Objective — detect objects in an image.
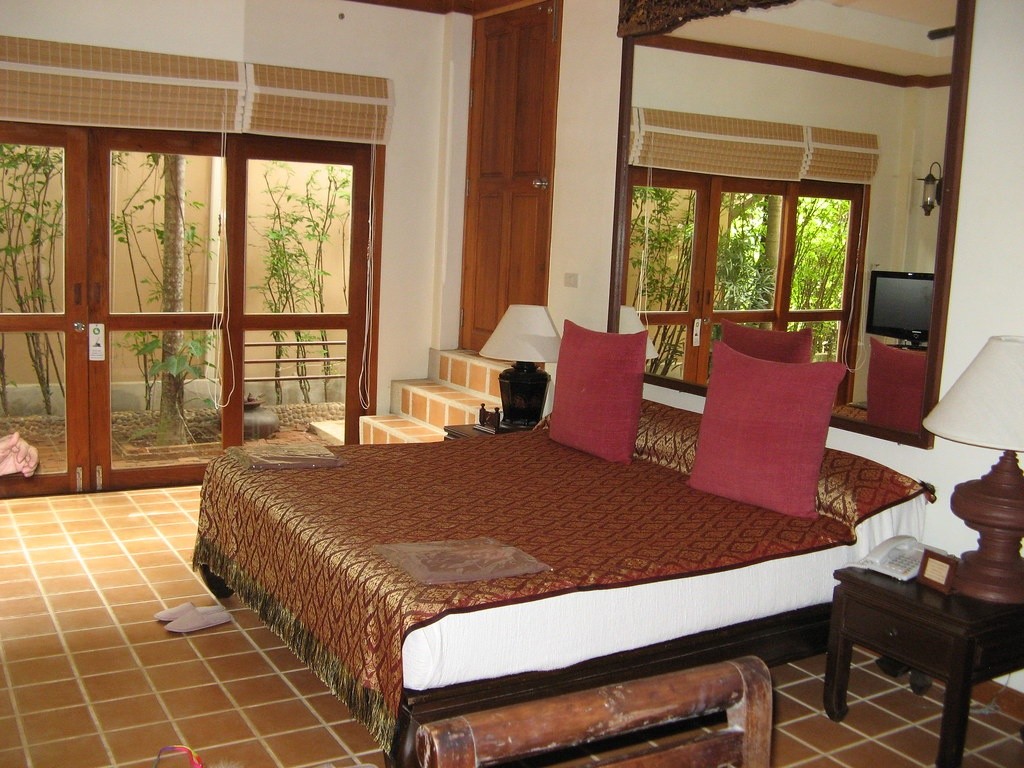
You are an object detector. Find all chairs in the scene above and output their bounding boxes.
[414,655,775,768]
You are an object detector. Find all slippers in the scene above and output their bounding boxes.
[163,608,234,633]
[154,601,226,621]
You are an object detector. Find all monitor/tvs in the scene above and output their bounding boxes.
[865,269,935,351]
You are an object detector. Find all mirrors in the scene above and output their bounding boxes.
[604,0,975,451]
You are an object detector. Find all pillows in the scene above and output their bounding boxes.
[718,316,814,363]
[867,334,926,434]
[548,318,648,469]
[685,339,847,521]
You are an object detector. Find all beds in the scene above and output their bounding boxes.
[194,397,938,768]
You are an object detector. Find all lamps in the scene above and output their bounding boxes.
[923,333,1024,604]
[478,303,561,429]
[916,160,942,218]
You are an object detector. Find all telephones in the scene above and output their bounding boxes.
[862,535,948,582]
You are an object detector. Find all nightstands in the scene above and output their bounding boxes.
[443,424,509,440]
[822,563,1023,768]
